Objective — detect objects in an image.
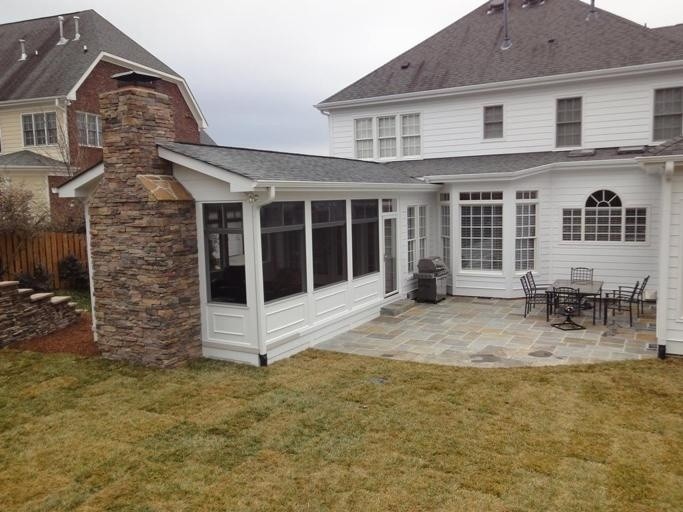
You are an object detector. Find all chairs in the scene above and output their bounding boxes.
[519,267,649,331]
